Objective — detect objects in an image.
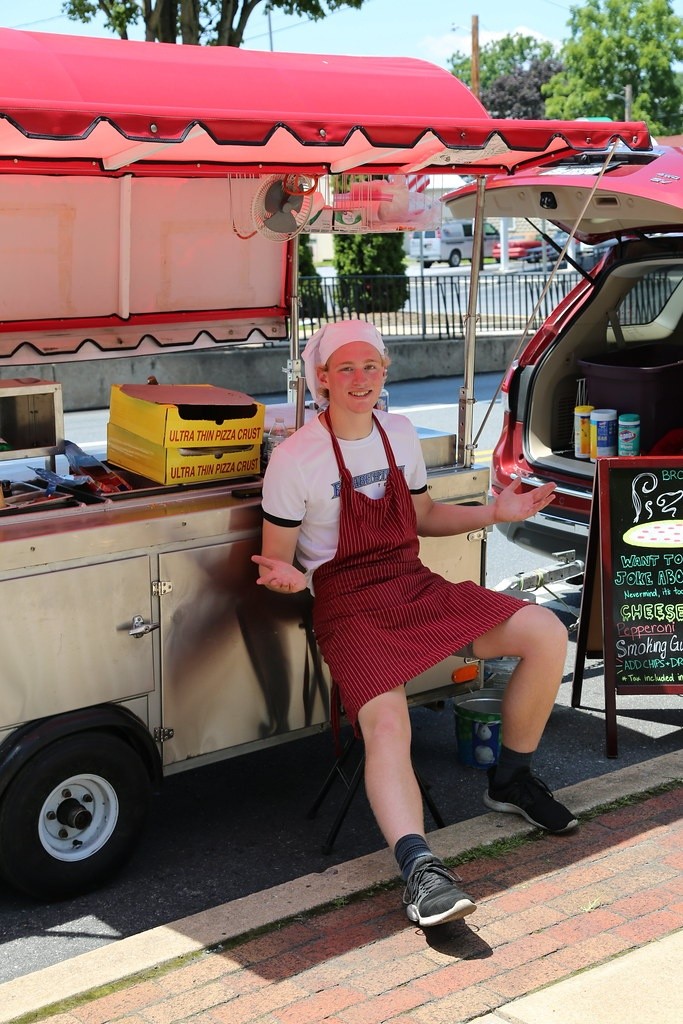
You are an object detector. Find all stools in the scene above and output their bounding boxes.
[308,672,447,855]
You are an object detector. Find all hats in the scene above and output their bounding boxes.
[301,319,385,411]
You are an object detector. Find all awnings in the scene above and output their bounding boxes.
[0,97,652,447]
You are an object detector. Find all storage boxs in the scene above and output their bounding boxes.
[107,421,261,486]
[111,382,265,448]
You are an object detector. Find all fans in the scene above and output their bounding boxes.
[250,174,313,243]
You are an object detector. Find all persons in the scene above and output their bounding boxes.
[251,318,579,927]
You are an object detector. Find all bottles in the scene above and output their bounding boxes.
[265,417,289,469]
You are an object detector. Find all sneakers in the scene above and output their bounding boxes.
[401,855,476,926]
[482,766,579,833]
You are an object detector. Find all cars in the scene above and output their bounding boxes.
[437,133,682,561]
[543,227,582,259]
[578,233,648,256]
[493,232,553,263]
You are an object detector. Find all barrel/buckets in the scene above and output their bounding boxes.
[452,688,508,769]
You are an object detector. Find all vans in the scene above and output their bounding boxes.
[409,219,500,269]
